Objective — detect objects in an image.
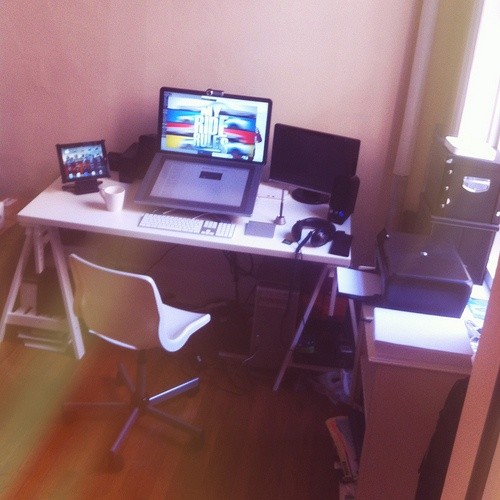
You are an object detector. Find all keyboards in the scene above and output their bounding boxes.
[137,210,237,239]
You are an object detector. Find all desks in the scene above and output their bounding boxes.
[0,166,361,392]
[323,269,493,500]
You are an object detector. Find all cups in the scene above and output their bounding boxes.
[99,186,125,211]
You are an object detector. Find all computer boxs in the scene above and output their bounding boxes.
[247,258,314,374]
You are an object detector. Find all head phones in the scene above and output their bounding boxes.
[291,217,337,248]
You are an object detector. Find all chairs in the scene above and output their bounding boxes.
[55,253,211,468]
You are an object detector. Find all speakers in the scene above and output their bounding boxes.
[327,175,361,225]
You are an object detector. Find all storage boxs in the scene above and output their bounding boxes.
[417,194,500,284]
[425,150,500,224]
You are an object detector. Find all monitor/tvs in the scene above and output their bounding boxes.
[56,139,112,196]
[267,123,361,204]
[156,87,275,171]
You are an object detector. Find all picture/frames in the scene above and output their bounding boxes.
[57,139,109,180]
